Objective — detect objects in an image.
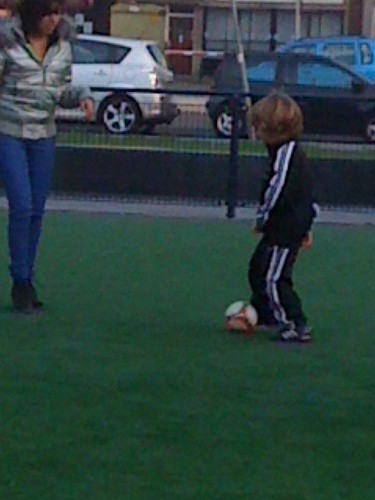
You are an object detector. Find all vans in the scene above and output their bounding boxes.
[273,37,375,83]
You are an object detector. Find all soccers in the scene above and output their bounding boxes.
[221,300,257,332]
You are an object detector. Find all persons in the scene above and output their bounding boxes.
[0,0,96,315]
[249,90,321,340]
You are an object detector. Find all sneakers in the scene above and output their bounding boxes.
[270,329,311,343]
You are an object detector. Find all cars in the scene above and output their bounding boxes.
[205,50,374,144]
[54,34,180,133]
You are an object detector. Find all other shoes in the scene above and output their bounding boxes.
[11,284,42,315]
